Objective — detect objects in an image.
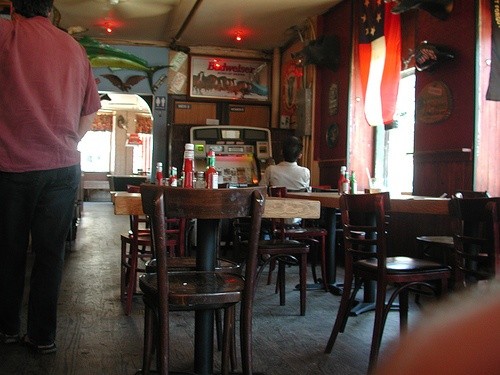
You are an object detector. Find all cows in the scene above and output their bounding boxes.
[286,16,342,71]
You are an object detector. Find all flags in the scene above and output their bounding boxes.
[358,0,401,126]
[486,0,500,101]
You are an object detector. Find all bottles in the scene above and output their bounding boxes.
[195,151,218,189]
[338,166,357,195]
[182,144,194,188]
[156,162,177,187]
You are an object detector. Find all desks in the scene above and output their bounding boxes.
[288,192,381,294]
[115,192,320,375]
[348,194,452,316]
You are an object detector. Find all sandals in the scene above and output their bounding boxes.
[22,334,57,354]
[0,334,19,346]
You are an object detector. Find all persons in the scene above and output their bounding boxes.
[259,136,310,228]
[0,0,102,354]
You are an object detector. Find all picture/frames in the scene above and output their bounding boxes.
[278,32,307,130]
[188,53,272,105]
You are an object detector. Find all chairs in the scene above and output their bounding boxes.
[121,166,500,375]
